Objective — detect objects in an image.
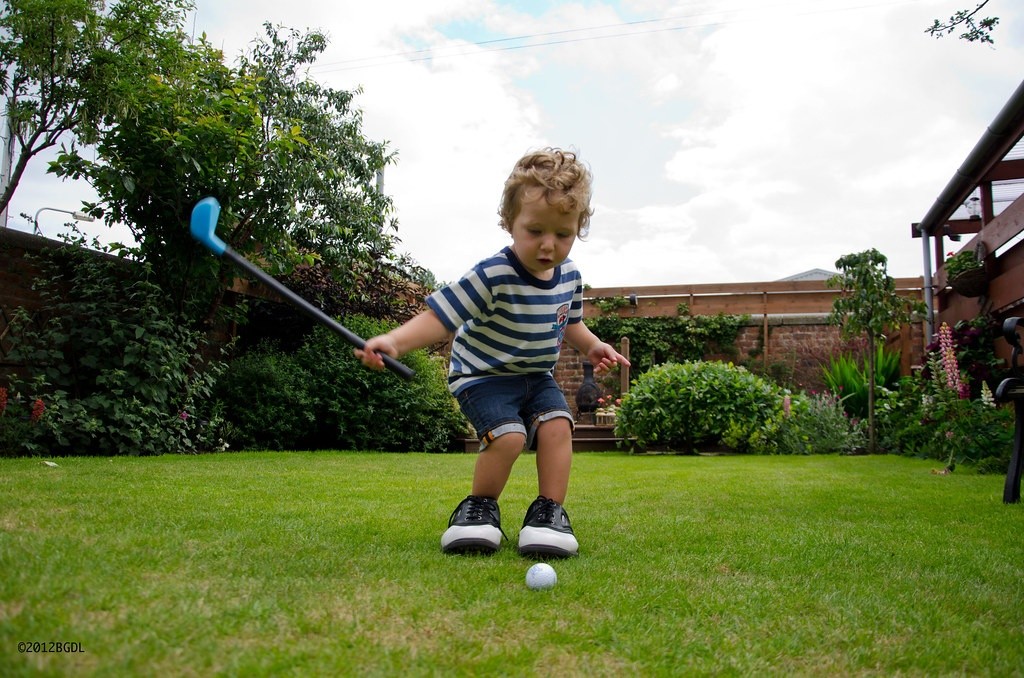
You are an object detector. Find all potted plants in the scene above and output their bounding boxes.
[947,250,988,297]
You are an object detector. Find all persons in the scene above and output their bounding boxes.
[353,147,631,559]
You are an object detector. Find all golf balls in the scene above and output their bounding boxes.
[523,562,557,593]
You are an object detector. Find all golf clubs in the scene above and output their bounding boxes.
[188,197,417,385]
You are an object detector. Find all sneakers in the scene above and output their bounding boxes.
[518,498,578,557]
[440,497,502,552]
